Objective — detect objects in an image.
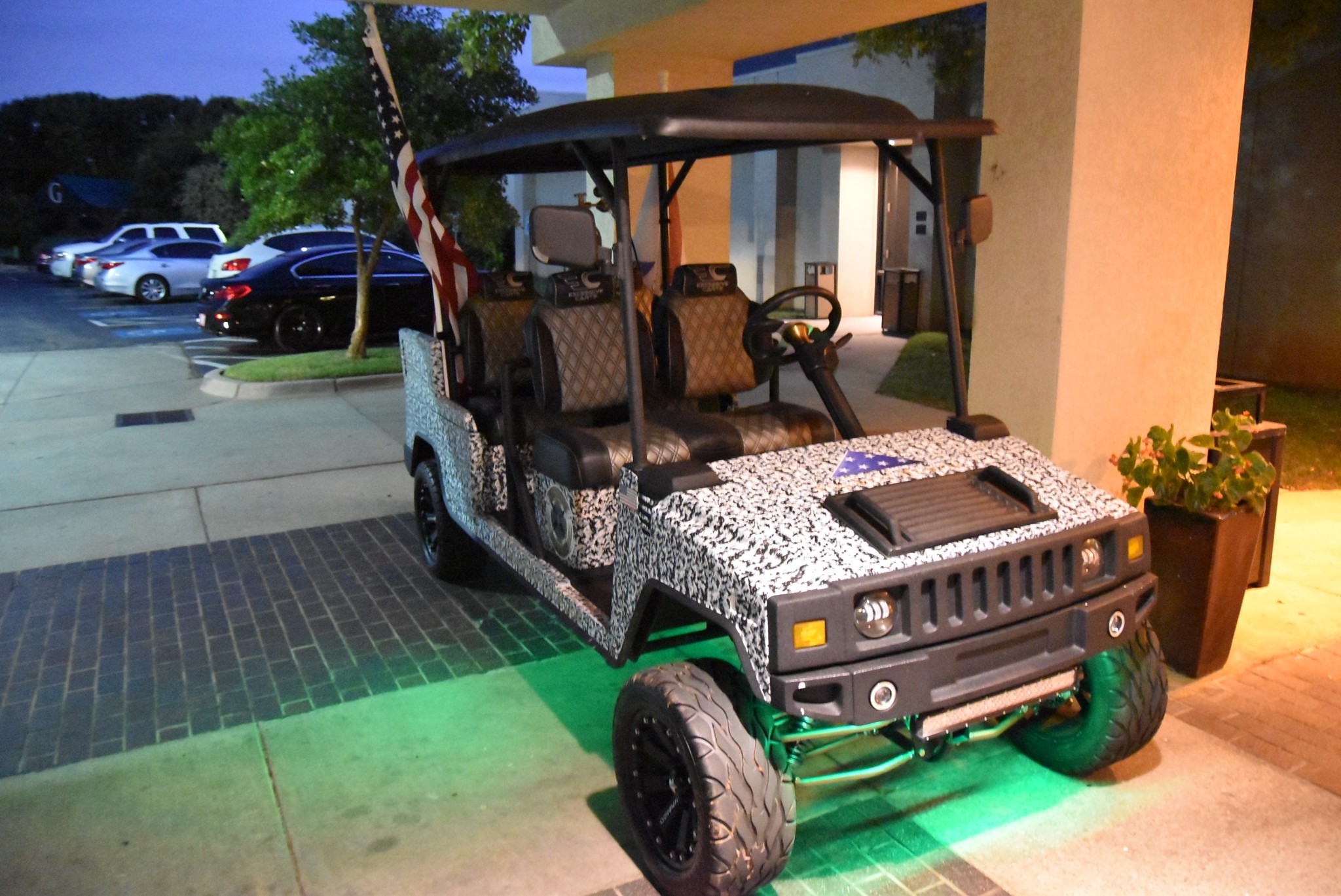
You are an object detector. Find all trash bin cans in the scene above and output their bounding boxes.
[879,268,920,333]
[804,261,835,318]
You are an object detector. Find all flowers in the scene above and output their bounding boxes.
[1109,407,1277,518]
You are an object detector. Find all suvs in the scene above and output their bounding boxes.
[51,223,228,286]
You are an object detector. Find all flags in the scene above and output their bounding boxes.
[361,4,485,323]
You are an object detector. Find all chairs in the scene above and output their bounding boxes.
[524,269,714,490]
[651,264,835,461]
[612,271,657,377]
[458,272,537,449]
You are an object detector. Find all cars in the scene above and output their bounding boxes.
[37,249,51,273]
[75,240,148,287]
[195,243,436,354]
[98,239,225,303]
[206,224,404,279]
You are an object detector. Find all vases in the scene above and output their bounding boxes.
[1144,494,1264,678]
